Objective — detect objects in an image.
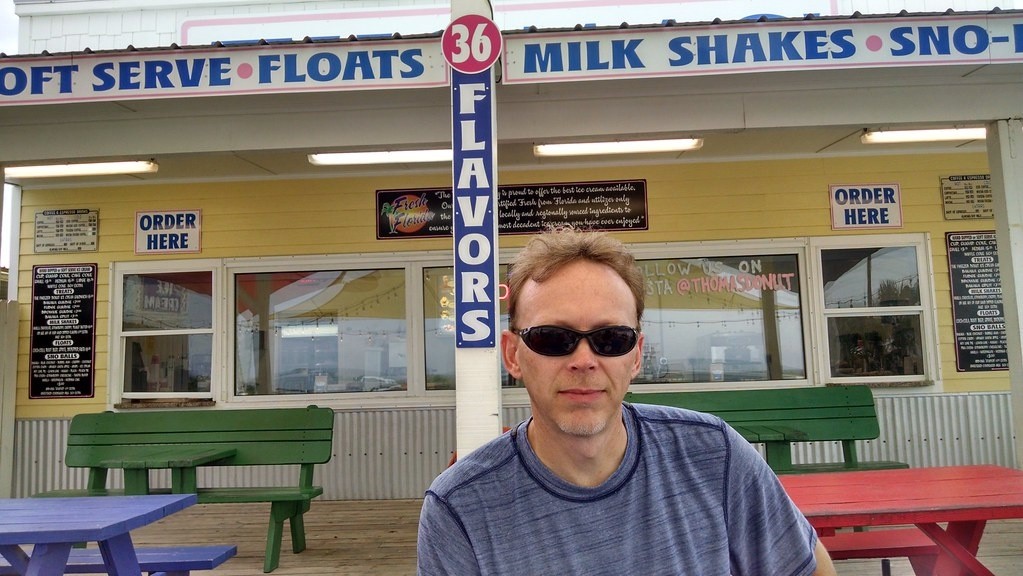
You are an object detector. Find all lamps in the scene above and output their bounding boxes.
[534,139,704,157]
[860,127,987,144]
[307,148,453,167]
[5,160,159,178]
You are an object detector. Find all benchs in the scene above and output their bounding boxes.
[623,383,909,534]
[30,405,336,573]
[820,528,946,576]
[0,545,237,576]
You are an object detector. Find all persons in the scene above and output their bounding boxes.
[416,222,836,576]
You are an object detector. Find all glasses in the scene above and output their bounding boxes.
[512,325,640,357]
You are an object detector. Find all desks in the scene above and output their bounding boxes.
[99,447,236,496]
[0,494,198,576]
[777,465,1023,576]
[730,423,808,473]
